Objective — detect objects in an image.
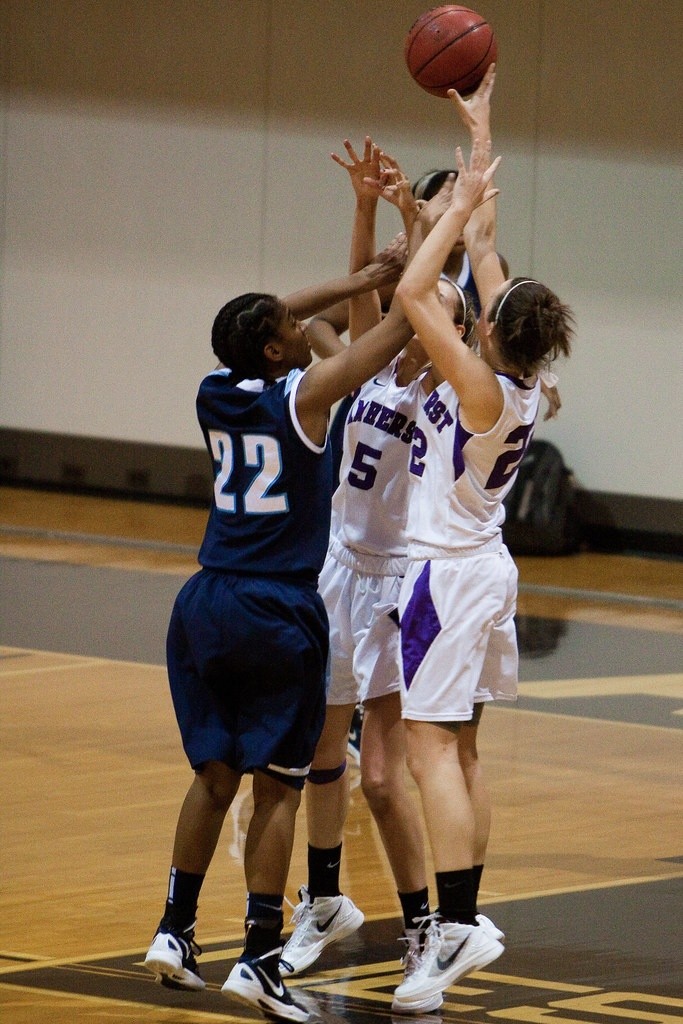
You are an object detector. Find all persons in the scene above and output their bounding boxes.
[309,169,562,767]
[395,63,571,1003]
[278,134,474,976]
[144,173,462,1022]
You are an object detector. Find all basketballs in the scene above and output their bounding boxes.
[405,4,499,98]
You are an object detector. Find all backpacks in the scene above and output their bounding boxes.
[501,440,569,550]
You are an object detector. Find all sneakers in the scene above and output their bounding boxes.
[346,703,364,763]
[278,885,364,976]
[474,914,504,940]
[221,959,324,1024]
[390,929,443,1013]
[144,925,206,991]
[394,912,505,1002]
